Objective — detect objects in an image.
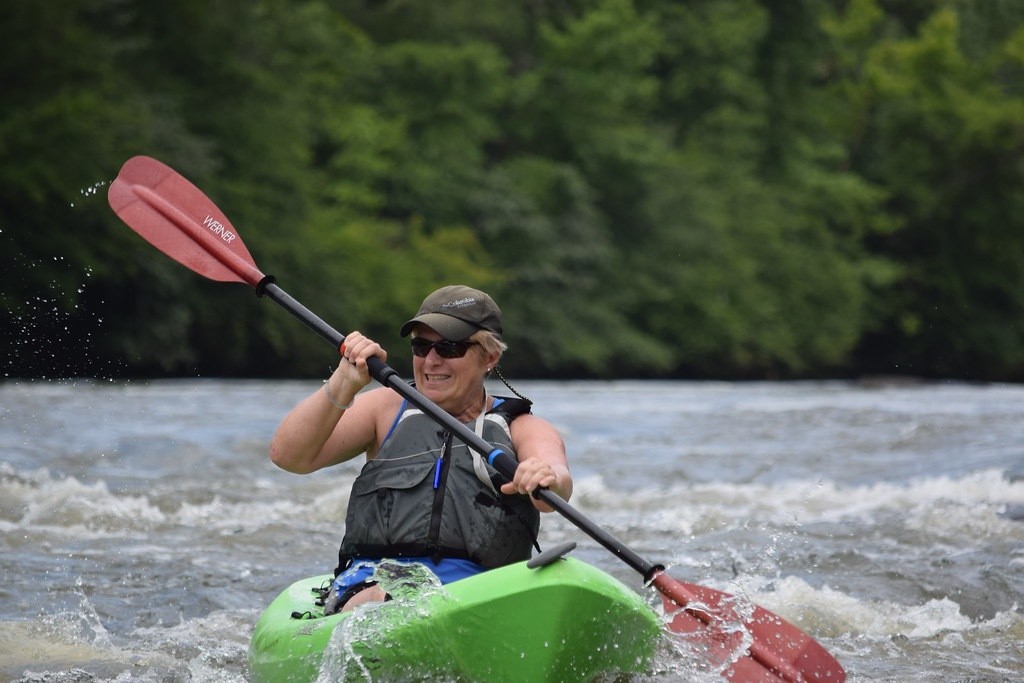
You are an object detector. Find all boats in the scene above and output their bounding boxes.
[247,541,666,683]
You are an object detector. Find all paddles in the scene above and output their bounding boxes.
[107,155,847,683]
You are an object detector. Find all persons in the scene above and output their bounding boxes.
[270,286,572,616]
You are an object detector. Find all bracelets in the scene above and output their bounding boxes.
[323,380,354,410]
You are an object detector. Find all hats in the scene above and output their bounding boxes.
[401,285,503,343]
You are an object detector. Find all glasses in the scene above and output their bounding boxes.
[409,338,480,359]
